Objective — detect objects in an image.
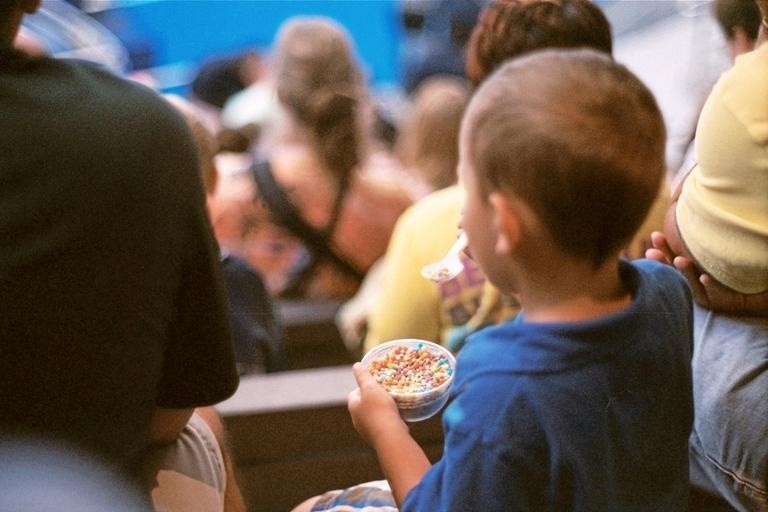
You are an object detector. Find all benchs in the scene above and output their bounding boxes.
[220,363,442,512]
[266,294,365,372]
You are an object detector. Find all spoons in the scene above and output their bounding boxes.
[421,230,469,283]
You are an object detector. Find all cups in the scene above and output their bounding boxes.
[360,338,456,423]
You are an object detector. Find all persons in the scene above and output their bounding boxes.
[1,1,768,512]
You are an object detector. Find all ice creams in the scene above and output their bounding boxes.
[369,341,453,422]
